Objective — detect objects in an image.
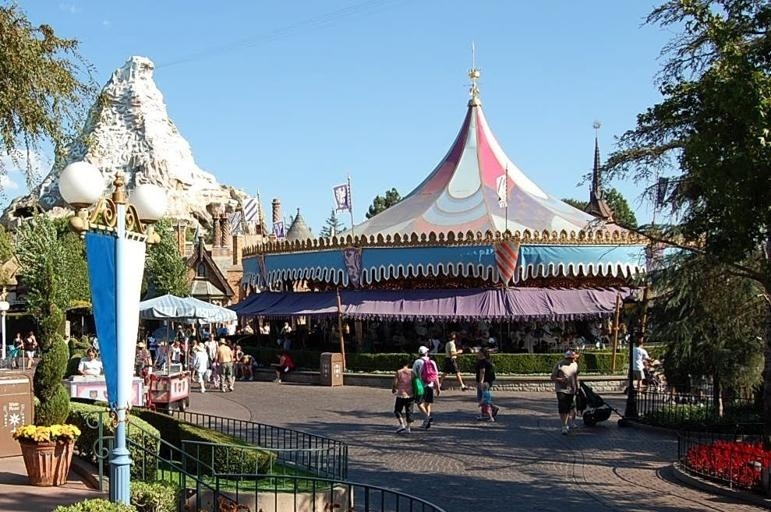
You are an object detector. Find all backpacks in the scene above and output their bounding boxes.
[420,358,437,382]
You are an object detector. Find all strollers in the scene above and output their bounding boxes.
[0,349,20,369]
[579,381,625,426]
[642,362,659,392]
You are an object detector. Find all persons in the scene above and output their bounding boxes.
[440,331,471,391]
[316,316,627,353]
[568,396,578,428]
[392,358,415,434]
[475,348,499,417]
[551,349,579,435]
[78,348,103,376]
[135,322,295,394]
[411,346,441,428]
[477,382,495,422]
[628,336,653,390]
[7,330,38,369]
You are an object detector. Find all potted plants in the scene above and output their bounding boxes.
[10,198,83,488]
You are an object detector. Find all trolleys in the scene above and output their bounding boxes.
[60,363,188,415]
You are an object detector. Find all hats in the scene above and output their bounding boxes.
[197,343,206,351]
[136,342,146,347]
[565,351,579,358]
[218,338,227,344]
[418,346,429,354]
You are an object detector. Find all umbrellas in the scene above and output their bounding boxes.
[139,291,220,376]
[182,295,238,325]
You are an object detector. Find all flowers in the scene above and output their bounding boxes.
[11,424,83,444]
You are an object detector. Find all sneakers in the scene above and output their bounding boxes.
[396,425,410,434]
[562,424,570,434]
[423,416,433,427]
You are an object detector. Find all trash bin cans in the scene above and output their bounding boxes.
[1,369,35,456]
[321,352,343,386]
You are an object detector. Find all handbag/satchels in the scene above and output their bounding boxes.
[414,376,424,402]
